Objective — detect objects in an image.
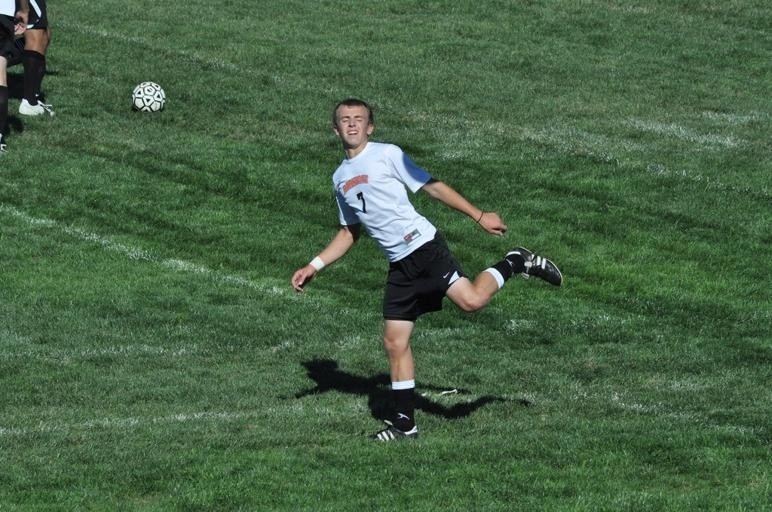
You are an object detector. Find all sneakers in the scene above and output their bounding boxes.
[505,246,563,287]
[18,98,56,117]
[368,424,418,445]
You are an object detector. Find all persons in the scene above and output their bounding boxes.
[289,98,564,445]
[1,0,30,152]
[7,0,56,118]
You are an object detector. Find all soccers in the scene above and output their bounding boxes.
[132,81,166,114]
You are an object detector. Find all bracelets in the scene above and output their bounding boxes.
[309,256,326,272]
[475,211,484,224]
